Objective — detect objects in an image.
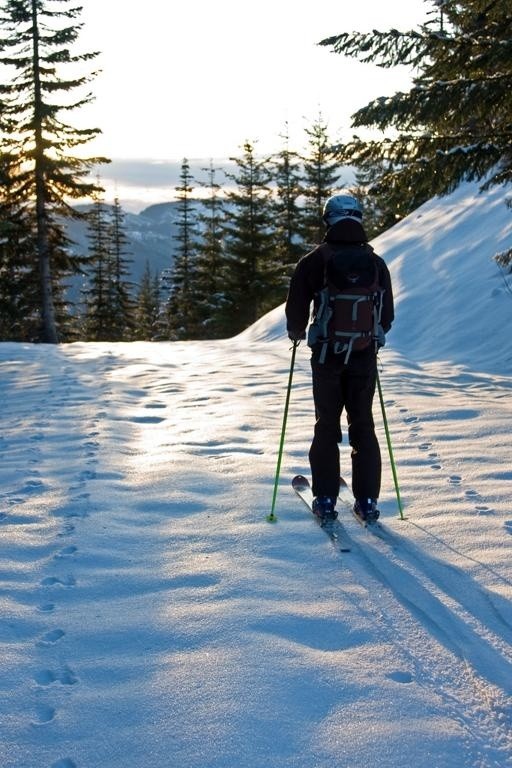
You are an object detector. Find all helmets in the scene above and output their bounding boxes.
[322,195,362,225]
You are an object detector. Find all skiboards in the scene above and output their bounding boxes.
[291,474,382,552]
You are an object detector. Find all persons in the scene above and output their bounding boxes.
[285,193,394,520]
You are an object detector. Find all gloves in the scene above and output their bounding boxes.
[288,330,306,340]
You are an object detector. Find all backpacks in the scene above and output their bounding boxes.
[311,244,384,357]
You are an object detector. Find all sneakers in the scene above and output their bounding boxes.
[312,496,338,520]
[353,498,380,521]
